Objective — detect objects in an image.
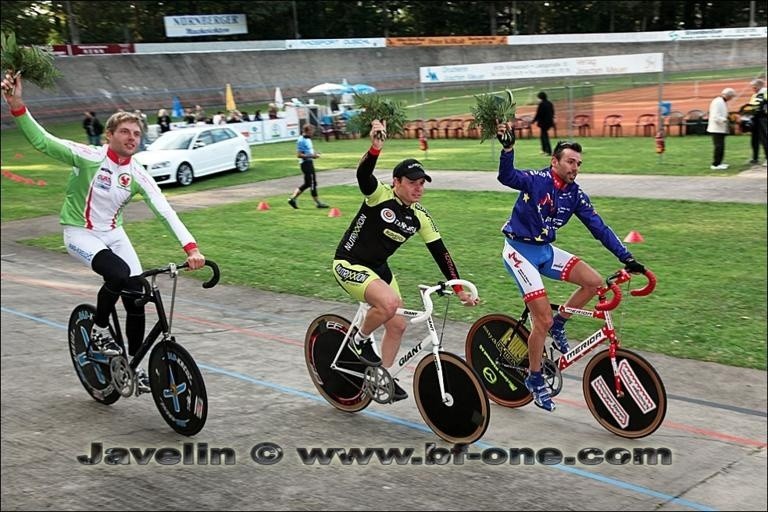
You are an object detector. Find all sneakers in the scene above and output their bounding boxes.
[721,164,729,167]
[379,378,408,399]
[288,198,298,208]
[711,165,728,169]
[749,160,759,163]
[348,333,383,367]
[90,324,124,355]
[134,368,152,393]
[762,160,767,167]
[317,203,330,208]
[525,376,556,411]
[549,323,571,354]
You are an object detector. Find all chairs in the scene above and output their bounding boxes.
[319,109,710,141]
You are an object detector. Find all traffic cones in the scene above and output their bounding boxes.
[326,207,342,217]
[255,200,269,211]
[620,229,644,242]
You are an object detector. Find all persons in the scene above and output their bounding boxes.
[740,78,767,163]
[329,92,340,113]
[134,109,148,151]
[0,66,206,393]
[331,116,479,397]
[494,117,645,410]
[155,103,281,132]
[82,110,103,146]
[526,91,557,155]
[706,89,736,169]
[286,123,330,209]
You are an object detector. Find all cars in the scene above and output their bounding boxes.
[132,126,251,187]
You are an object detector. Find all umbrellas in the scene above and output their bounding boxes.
[274,86,285,113]
[171,92,185,122]
[308,80,353,105]
[225,83,236,114]
[352,84,378,95]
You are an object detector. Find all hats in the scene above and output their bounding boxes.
[393,159,432,182]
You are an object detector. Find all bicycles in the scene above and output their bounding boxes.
[66,260,221,436]
[462,263,669,439]
[301,277,489,443]
